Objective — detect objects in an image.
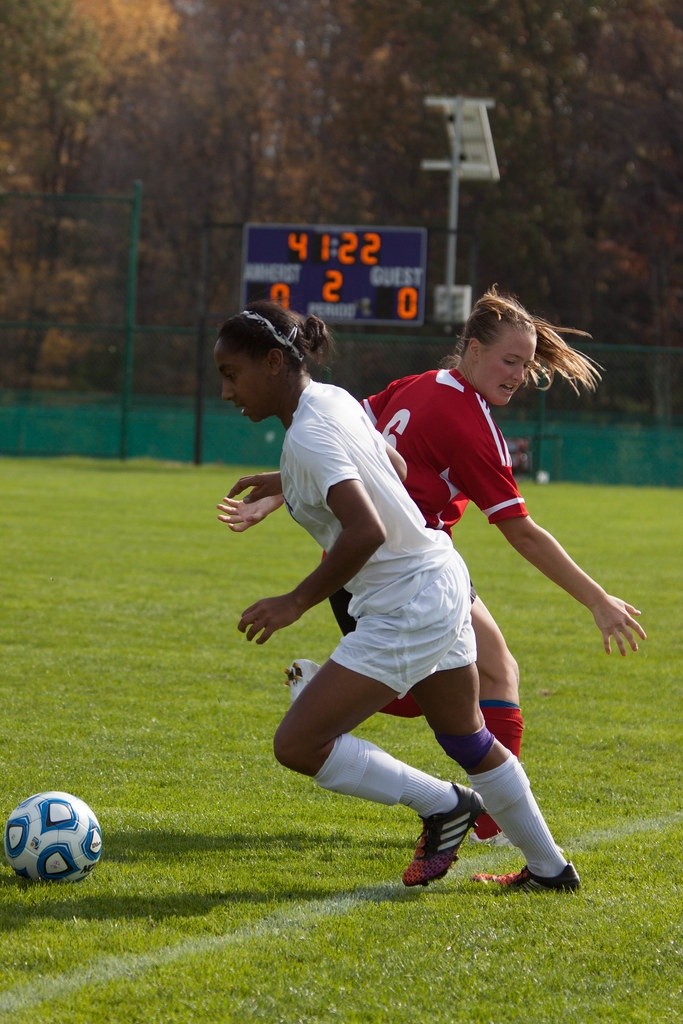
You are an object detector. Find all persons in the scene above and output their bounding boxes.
[212,301,583,893]
[215,292,647,845]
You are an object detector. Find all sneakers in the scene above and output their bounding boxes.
[471,860,582,893]
[283,657,323,708]
[402,781,486,887]
[468,810,503,845]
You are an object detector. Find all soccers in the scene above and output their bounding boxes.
[7,791,106,882]
